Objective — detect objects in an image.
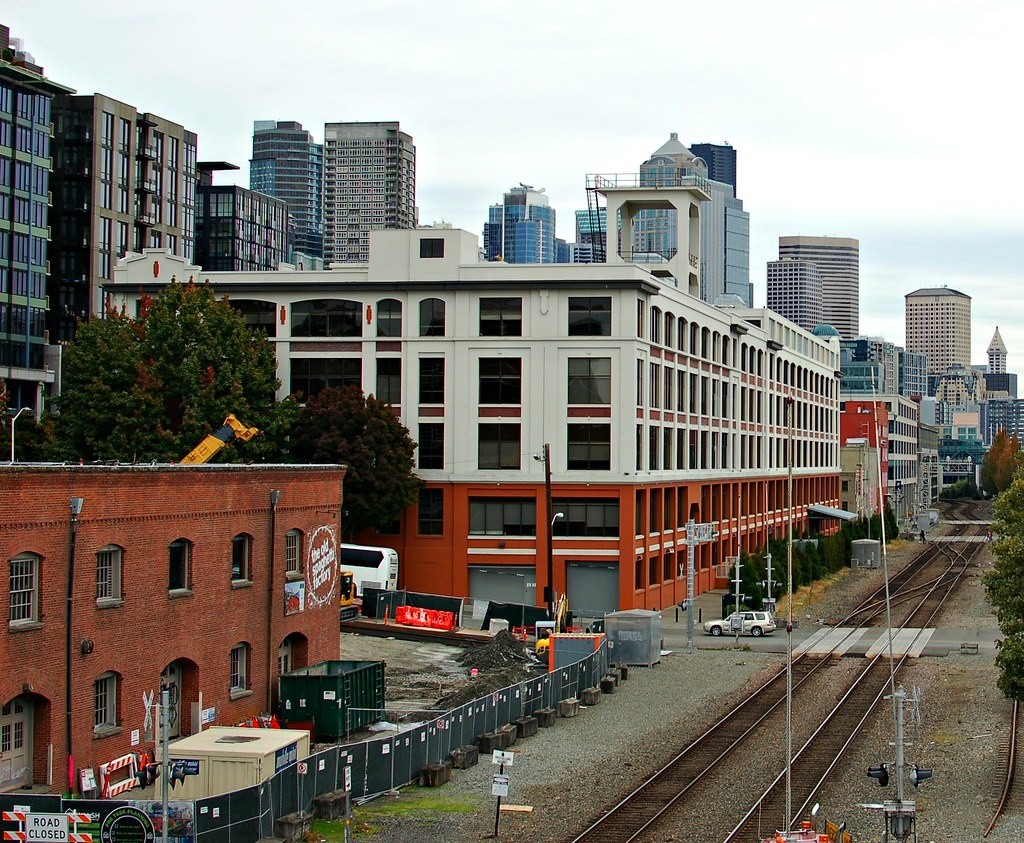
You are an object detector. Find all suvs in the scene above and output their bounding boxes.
[702,610,777,637]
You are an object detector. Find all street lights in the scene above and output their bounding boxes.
[548,511,564,622]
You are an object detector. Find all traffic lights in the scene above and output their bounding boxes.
[908,764,934,788]
[867,763,889,786]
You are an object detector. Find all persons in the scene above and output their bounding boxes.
[986,531,992,541]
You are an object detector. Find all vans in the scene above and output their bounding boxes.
[340,543,400,608]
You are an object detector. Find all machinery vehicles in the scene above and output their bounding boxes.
[340,572,361,623]
